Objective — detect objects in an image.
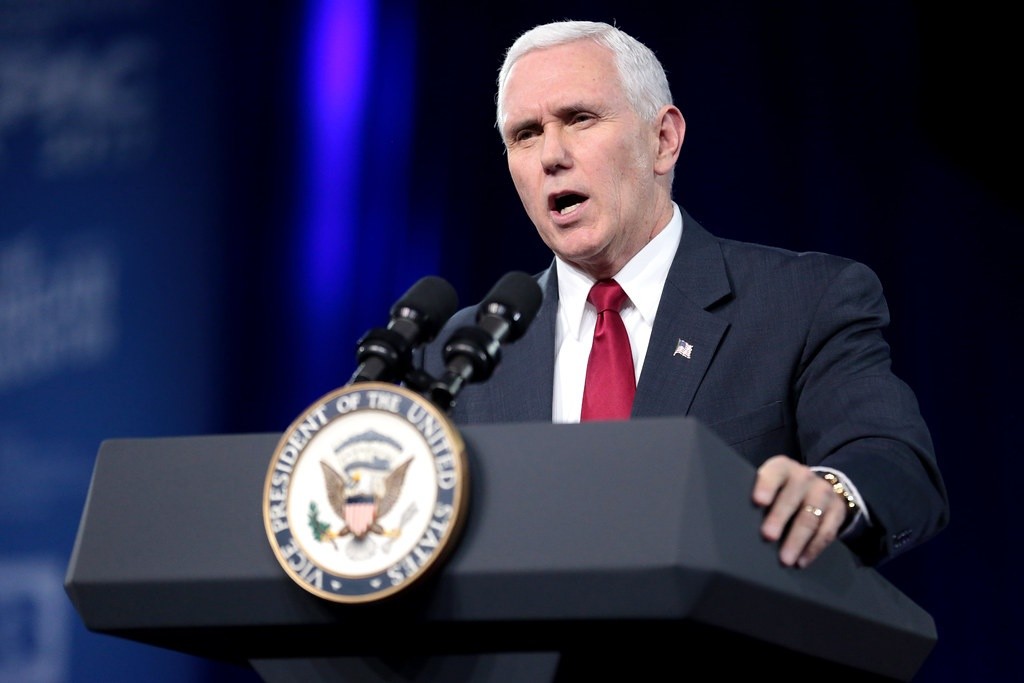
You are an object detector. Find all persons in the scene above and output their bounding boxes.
[396,15,949,573]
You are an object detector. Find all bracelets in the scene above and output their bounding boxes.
[808,465,857,519]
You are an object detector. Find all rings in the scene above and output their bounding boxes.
[800,502,824,519]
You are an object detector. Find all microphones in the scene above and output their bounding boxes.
[432,271,543,411]
[346,276,457,384]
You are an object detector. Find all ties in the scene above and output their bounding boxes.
[579,279,637,424]
